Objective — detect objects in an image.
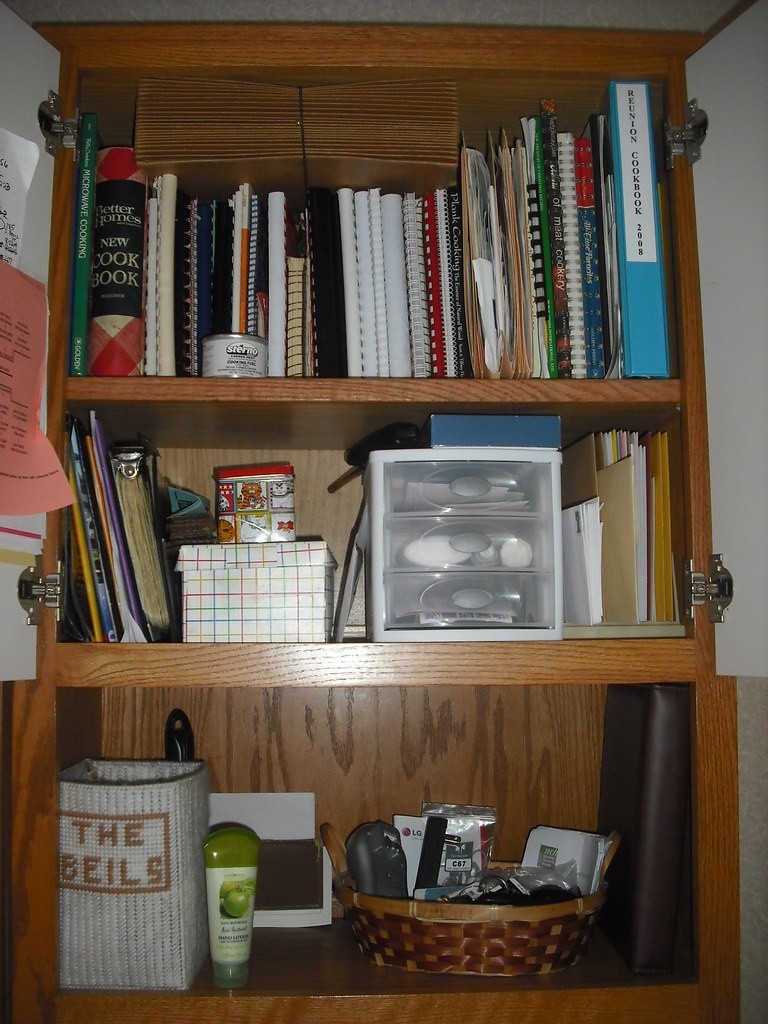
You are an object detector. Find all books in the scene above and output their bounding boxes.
[72,75,670,380]
[560,427,680,623]
[61,409,178,644]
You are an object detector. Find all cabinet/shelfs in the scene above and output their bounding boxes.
[359,448,564,644]
[9,18,741,1024]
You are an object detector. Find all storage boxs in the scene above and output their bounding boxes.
[423,412,562,446]
[173,538,337,642]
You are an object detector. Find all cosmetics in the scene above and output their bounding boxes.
[204,827,261,981]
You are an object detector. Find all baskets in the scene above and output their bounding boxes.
[320,822,621,977]
